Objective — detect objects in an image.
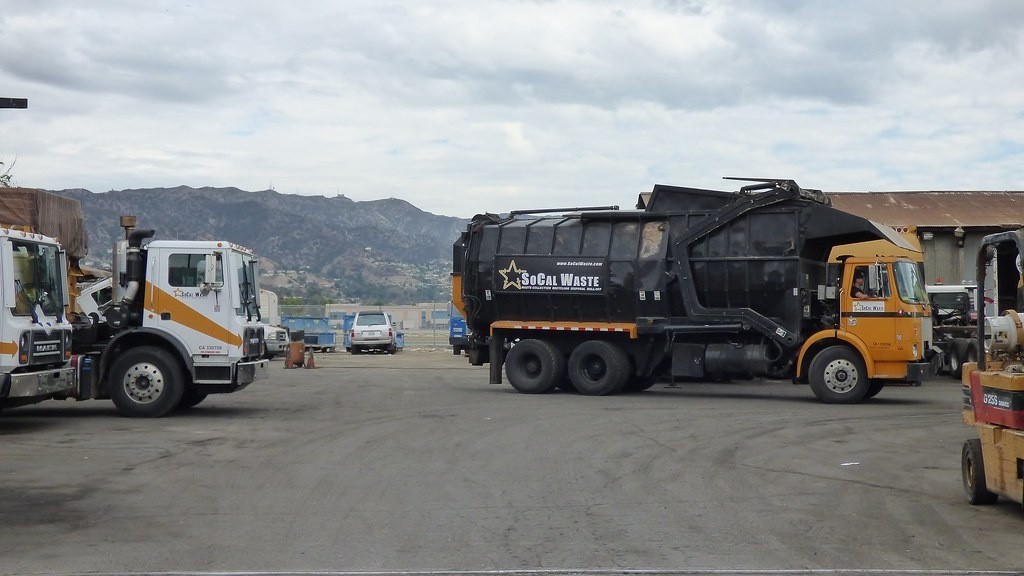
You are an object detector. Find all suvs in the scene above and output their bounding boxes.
[347,309,398,355]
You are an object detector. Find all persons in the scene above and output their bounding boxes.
[851,272,879,299]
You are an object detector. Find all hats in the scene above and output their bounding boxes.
[854,271,866,279]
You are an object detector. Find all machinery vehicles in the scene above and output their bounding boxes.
[959,225,1024,514]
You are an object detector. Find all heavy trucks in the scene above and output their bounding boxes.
[924,280,993,380]
[448,175,946,407]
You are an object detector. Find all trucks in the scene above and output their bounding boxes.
[448,300,474,355]
[0,185,293,420]
